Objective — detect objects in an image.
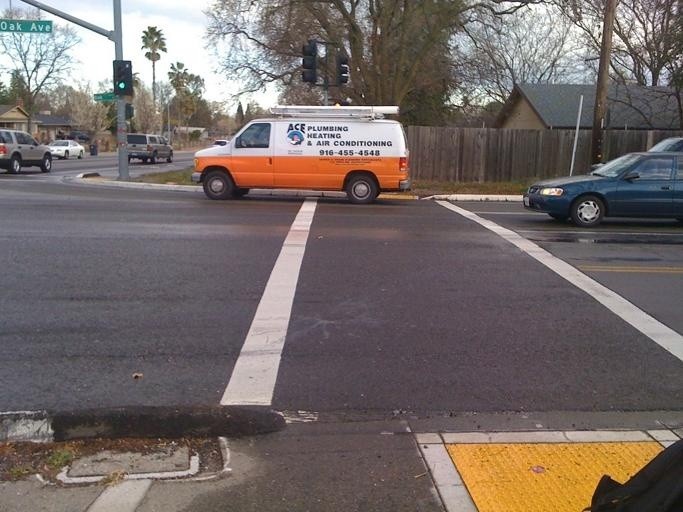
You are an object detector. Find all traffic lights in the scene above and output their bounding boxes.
[302,38,349,83]
[114,59,134,96]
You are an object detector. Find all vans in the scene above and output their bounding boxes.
[127,133,173,164]
[191,105,410,204]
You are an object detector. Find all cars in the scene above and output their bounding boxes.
[211,140,230,147]
[523,136,683,227]
[0,129,85,173]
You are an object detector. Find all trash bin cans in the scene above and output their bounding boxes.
[90,144,98,156]
